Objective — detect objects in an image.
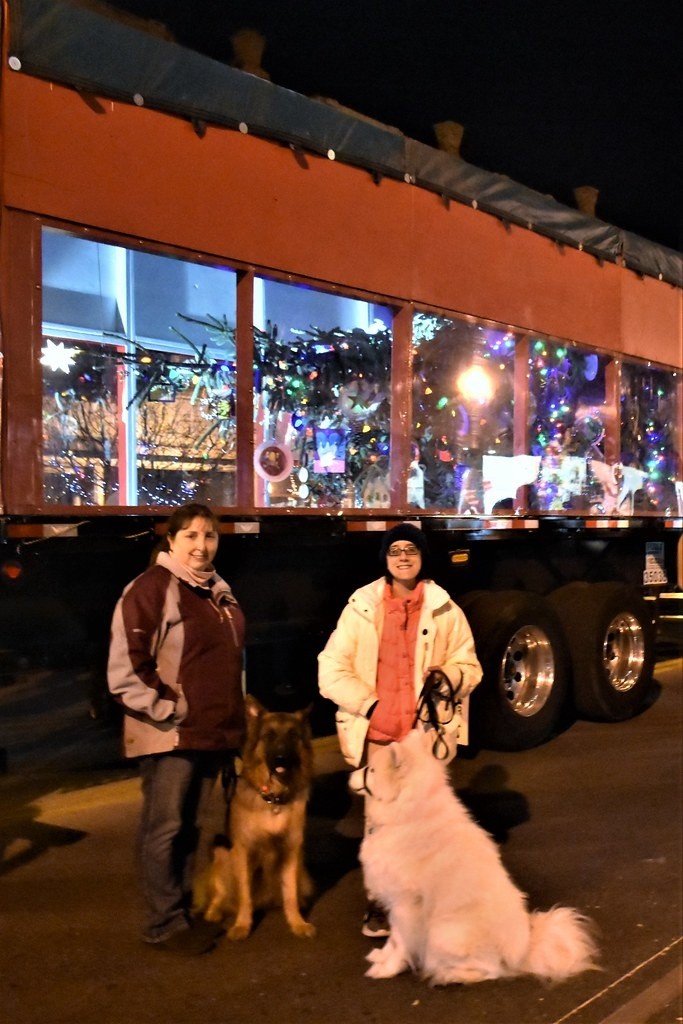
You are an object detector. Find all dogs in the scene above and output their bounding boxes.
[347,729,604,988]
[199,693,319,940]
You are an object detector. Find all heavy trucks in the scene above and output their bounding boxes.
[3,1,683,771]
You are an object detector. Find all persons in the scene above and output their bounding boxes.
[106,502,249,958]
[315,522,482,938]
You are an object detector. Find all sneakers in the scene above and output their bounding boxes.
[362,911,390,937]
[159,927,214,955]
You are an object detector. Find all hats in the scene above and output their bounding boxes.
[380,523,431,566]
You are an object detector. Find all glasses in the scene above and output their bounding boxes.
[386,546,420,557]
